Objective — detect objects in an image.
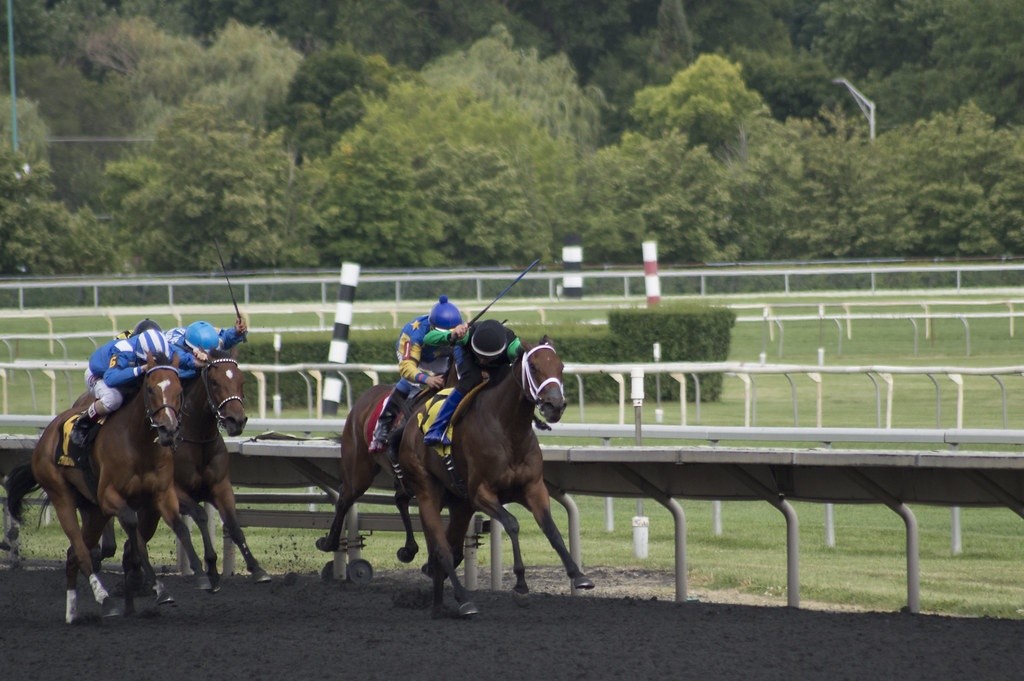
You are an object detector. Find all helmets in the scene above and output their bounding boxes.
[470,319,507,356]
[428,295,464,332]
[133,318,163,334]
[133,329,170,361]
[184,320,219,350]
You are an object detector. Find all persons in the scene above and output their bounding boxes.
[69,318,247,447]
[368,294,524,452]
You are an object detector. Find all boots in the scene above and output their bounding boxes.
[69,400,109,449]
[367,386,409,453]
[422,389,464,445]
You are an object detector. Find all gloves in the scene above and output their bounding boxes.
[143,349,157,372]
[235,315,247,335]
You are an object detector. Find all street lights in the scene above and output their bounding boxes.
[832,78,876,139]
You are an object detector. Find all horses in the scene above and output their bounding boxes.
[314,334,597,622]
[4,349,274,626]
